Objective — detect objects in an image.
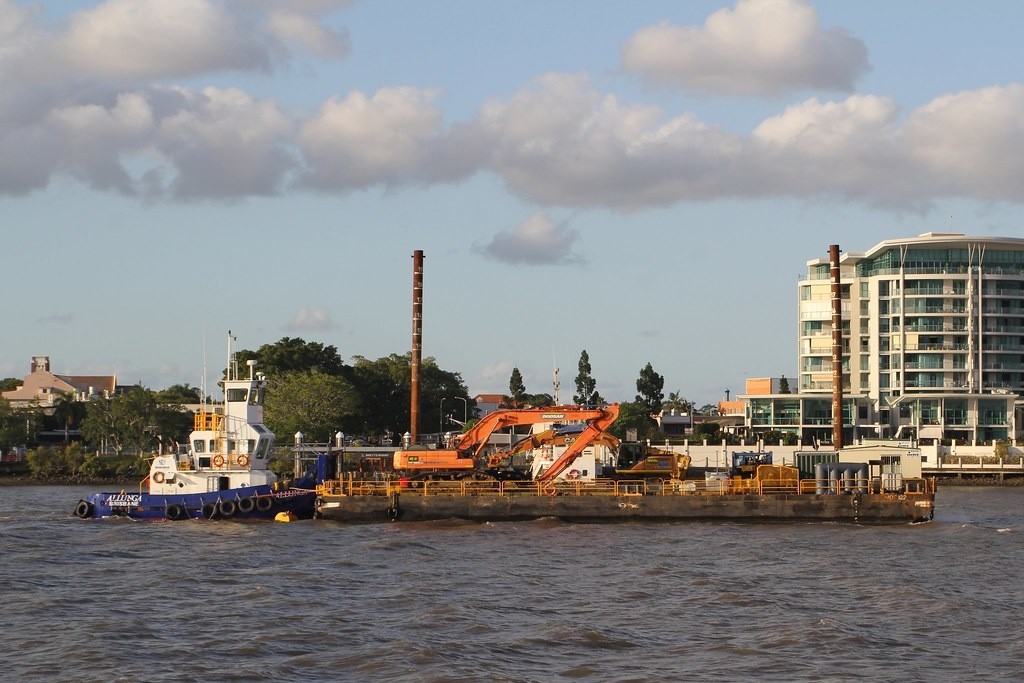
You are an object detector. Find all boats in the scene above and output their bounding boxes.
[73,325,339,526]
[310,463,939,521]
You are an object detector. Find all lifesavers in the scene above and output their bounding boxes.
[569,469,580,480]
[153,472,165,483]
[213,455,224,467]
[545,484,557,496]
[236,454,248,466]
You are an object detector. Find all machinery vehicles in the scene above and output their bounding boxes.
[729,449,772,482]
[615,439,692,481]
[389,402,622,487]
